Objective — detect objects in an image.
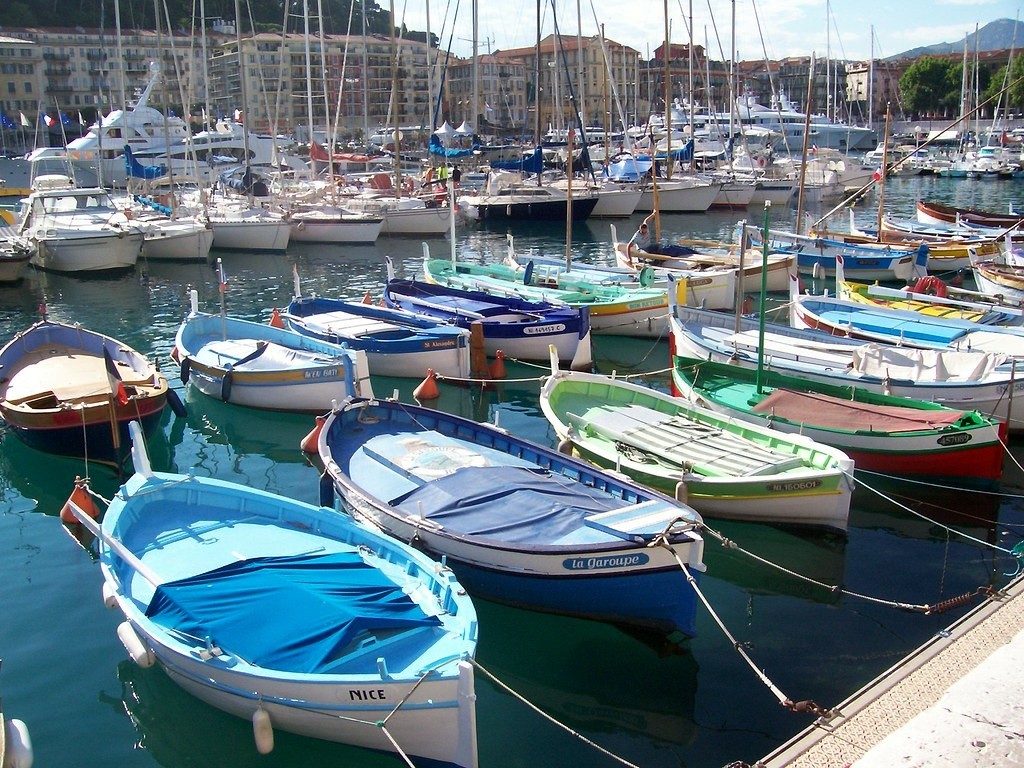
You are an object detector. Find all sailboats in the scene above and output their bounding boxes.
[185,0,294,255]
[441,0,1024,220]
[362,0,451,238]
[0,0,149,284]
[262,0,384,245]
[98,0,216,263]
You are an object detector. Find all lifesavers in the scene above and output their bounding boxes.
[913,276,947,297]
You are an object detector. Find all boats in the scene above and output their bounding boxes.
[316,354,707,638]
[848,203,1024,272]
[667,272,1024,431]
[0,304,169,470]
[538,345,856,531]
[609,224,800,293]
[668,331,1006,478]
[736,219,929,284]
[789,273,1024,360]
[422,242,678,340]
[835,254,1024,331]
[62,62,311,183]
[967,233,1024,306]
[67,420,481,768]
[660,88,875,151]
[491,234,742,309]
[380,255,593,370]
[171,289,375,417]
[286,261,470,388]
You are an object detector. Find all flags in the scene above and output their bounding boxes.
[568,121,576,138]
[217,108,223,121]
[235,109,242,122]
[78,112,86,126]
[812,142,819,153]
[201,109,207,120]
[59,110,72,127]
[873,166,881,181]
[188,114,192,122]
[39,111,55,126]
[169,109,174,116]
[0,114,16,129]
[20,113,33,127]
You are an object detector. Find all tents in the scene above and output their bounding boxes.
[455,121,474,148]
[433,119,457,147]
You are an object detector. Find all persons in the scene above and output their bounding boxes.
[625,209,657,264]
[421,167,436,190]
[452,165,462,188]
[435,163,447,188]
